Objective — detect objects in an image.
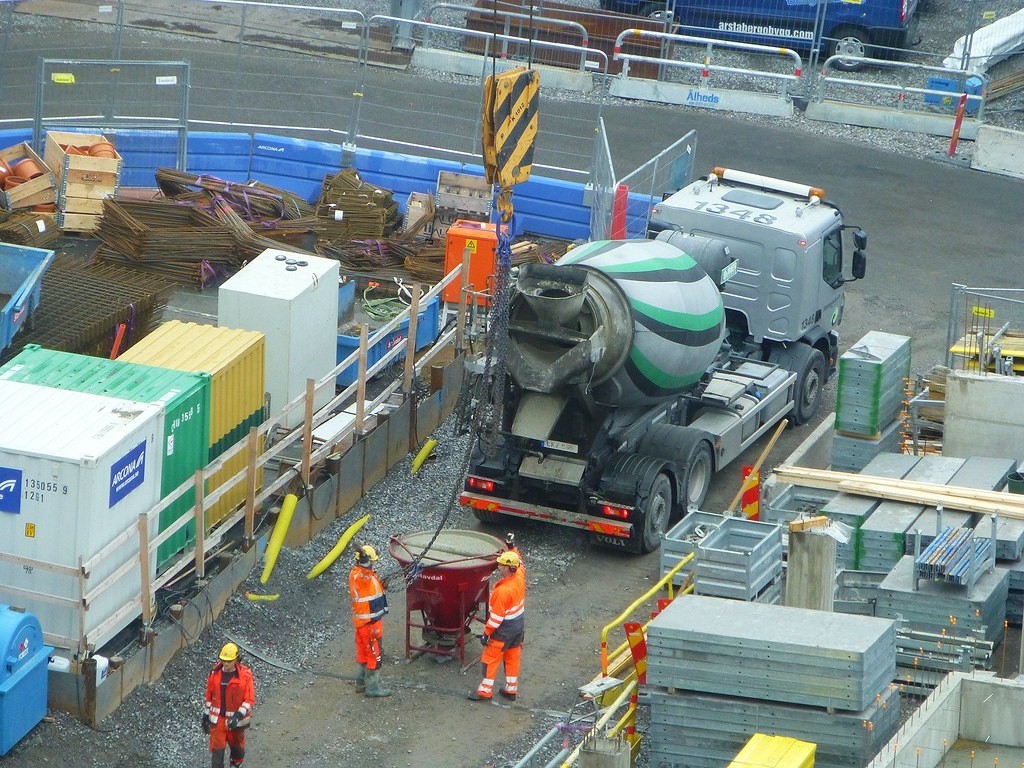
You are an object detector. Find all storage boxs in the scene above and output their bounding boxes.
[436,169,496,215]
[404,191,492,240]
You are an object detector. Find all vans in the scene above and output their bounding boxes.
[600,0,922,71]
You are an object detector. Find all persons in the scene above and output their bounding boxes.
[201,644,254,768]
[349,542,393,697]
[467,533,526,701]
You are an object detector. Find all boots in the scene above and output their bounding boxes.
[364,668,391,697]
[354,662,367,693]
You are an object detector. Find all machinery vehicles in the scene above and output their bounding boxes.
[461,165,868,556]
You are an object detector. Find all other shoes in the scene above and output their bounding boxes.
[468,691,492,700]
[229,758,243,768]
[499,688,516,700]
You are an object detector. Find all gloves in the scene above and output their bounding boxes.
[201,714,210,734]
[504,532,515,549]
[481,635,488,645]
[227,711,243,730]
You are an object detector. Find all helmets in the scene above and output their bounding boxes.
[497,551,520,565]
[218,643,238,661]
[355,545,378,561]
